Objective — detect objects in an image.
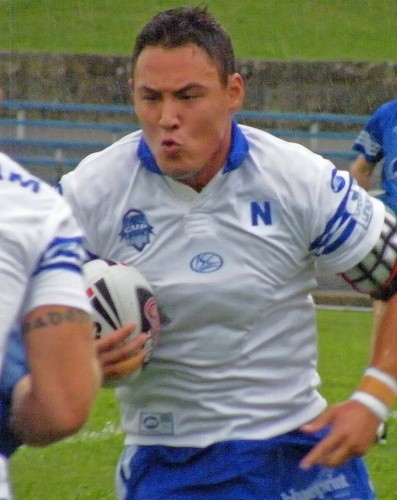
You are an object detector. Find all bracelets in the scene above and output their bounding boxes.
[350,368,397,421]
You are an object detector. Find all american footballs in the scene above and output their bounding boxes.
[82,258,160,389]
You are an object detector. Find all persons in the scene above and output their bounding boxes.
[0,152,102,500]
[53,1,397,500]
[350,98,397,445]
